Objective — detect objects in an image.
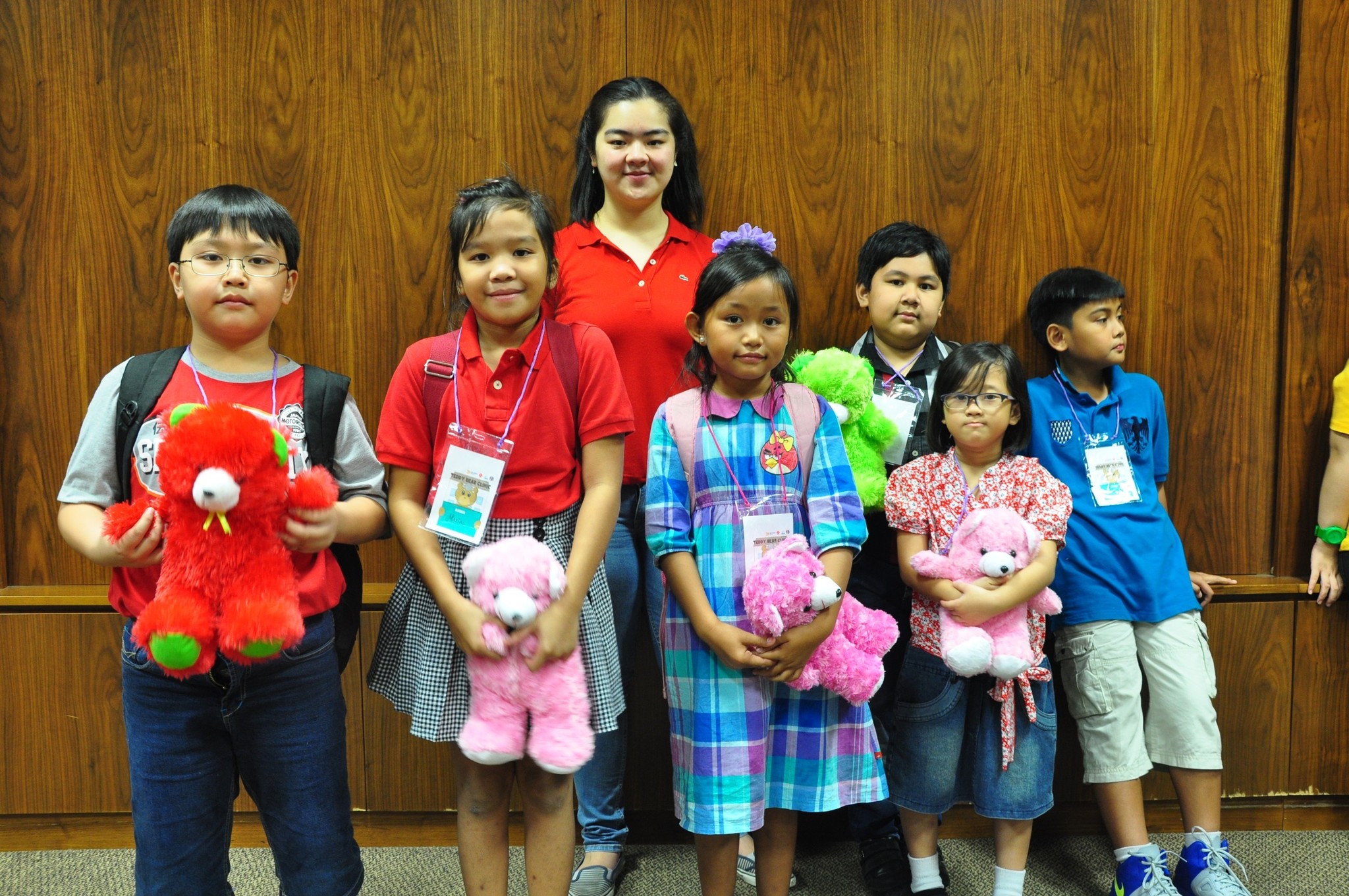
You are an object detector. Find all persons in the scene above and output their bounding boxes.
[885,341,1074,896]
[1306,356,1349,607]
[538,74,807,896]
[838,225,969,895]
[1016,268,1253,896]
[58,186,389,896]
[373,177,628,895]
[645,224,891,896]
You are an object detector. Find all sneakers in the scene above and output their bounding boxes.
[1172,840,1255,896]
[1107,844,1185,896]
[566,847,628,895]
[734,852,798,890]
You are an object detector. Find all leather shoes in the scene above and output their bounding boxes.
[905,843,950,891]
[857,836,904,893]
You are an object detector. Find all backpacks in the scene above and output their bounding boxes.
[114,346,362,685]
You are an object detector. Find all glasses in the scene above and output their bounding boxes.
[176,251,293,280]
[938,392,1016,413]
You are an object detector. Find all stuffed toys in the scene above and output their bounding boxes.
[455,534,597,776]
[740,531,901,707]
[909,504,1066,682]
[783,346,903,514]
[102,401,337,684]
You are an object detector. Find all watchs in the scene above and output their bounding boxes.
[1314,524,1347,546]
